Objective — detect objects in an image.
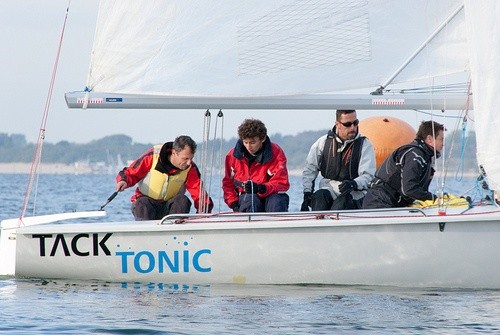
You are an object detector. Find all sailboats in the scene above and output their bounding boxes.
[0,0,499,294]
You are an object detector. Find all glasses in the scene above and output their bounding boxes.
[338,119,359,128]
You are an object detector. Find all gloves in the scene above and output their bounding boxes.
[339,179,357,195]
[304,191,315,208]
[243,182,266,194]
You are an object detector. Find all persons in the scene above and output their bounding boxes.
[220,119,290,213]
[299,109,377,212]
[116,135,213,222]
[361,120,448,209]
